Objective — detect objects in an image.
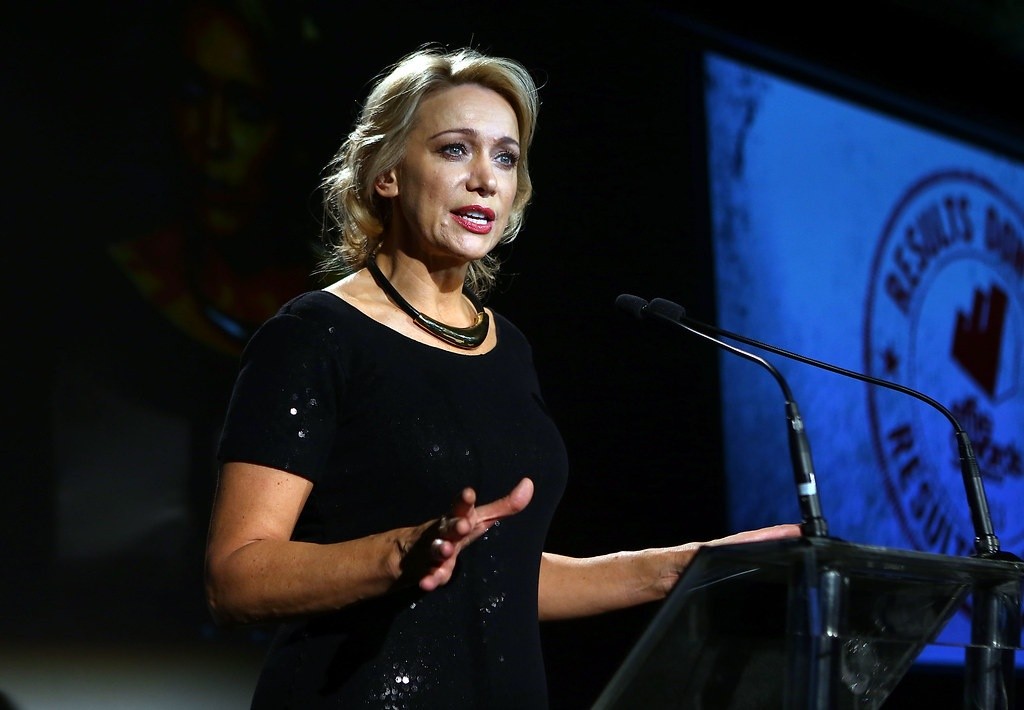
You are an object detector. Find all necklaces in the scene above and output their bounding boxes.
[367,241,490,350]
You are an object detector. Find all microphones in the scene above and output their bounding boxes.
[613,294,828,539]
[649,299,1019,560]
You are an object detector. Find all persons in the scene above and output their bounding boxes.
[204,50,802,710]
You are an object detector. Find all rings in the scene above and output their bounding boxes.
[439,517,449,536]
